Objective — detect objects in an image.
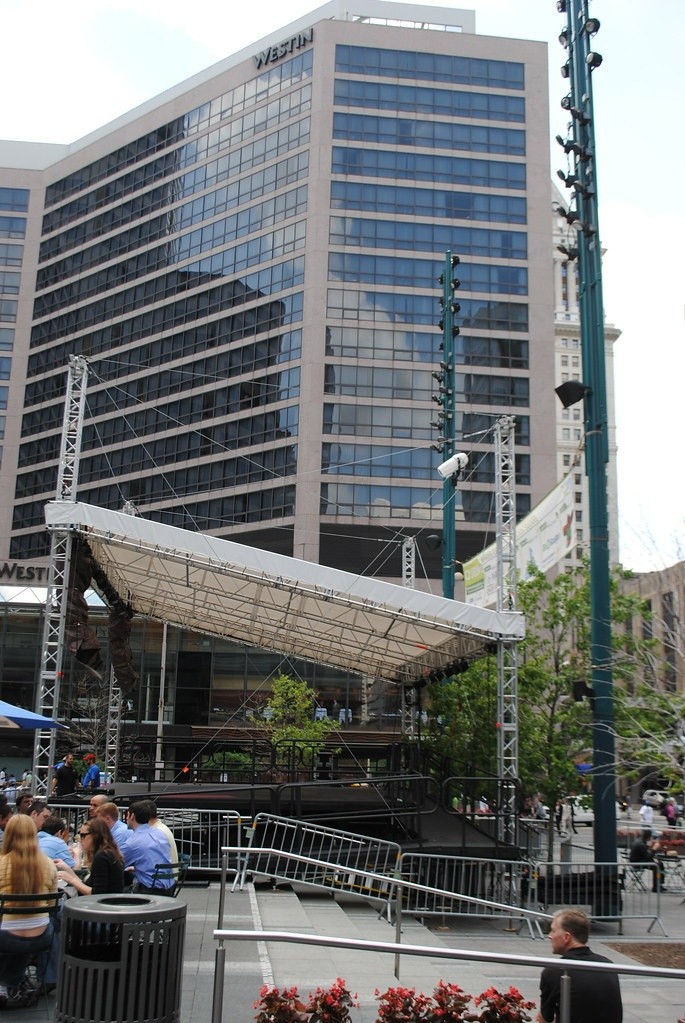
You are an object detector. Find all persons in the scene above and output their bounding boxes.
[82,753,101,793]
[0,767,33,802]
[0,795,179,1006]
[453,789,578,834]
[49,753,78,797]
[0,811,63,1003]
[331,699,340,721]
[532,909,623,1023]
[638,789,685,831]
[630,829,669,893]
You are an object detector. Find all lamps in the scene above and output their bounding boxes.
[412,642,497,689]
[435,453,468,490]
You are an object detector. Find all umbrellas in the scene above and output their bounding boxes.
[0,699,70,729]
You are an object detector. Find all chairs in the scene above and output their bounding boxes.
[620,851,685,895]
[0,853,192,1020]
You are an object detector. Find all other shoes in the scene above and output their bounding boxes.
[37,983,58,996]
[652,885,669,893]
[0,985,9,1006]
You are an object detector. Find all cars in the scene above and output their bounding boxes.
[642,790,685,816]
[542,794,622,826]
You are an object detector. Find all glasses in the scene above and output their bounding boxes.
[79,831,92,841]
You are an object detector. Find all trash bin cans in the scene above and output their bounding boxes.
[54,893,188,1023]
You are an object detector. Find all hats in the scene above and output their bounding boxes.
[83,753,95,761]
